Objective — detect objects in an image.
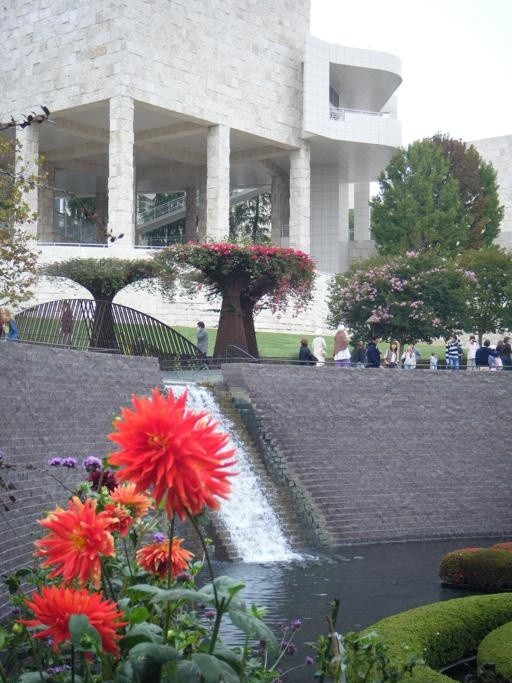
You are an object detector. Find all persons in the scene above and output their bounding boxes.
[4,306,18,339]
[400,345,417,369]
[333,330,352,367]
[356,339,367,368]
[366,336,381,368]
[311,330,327,367]
[59,300,74,343]
[195,321,209,370]
[298,338,312,365]
[410,343,420,363]
[445,332,512,371]
[384,341,398,368]
[428,352,438,369]
